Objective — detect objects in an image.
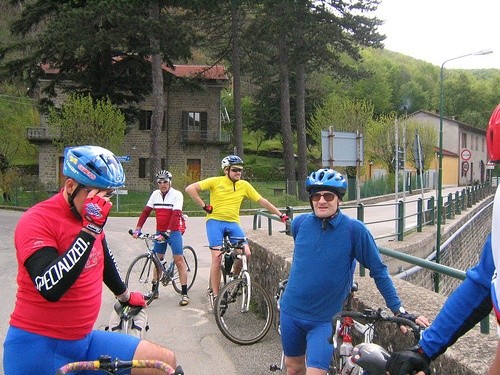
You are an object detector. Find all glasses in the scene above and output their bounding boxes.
[308,193,334,201]
[157,180,168,183]
[230,168,241,172]
[78,184,114,199]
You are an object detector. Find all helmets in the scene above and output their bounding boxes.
[63,145,126,188]
[222,155,244,169]
[486,103,500,162]
[156,170,173,179]
[306,168,347,196]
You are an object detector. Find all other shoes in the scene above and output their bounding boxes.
[237,284,243,295]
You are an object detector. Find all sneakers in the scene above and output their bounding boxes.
[179,295,189,305]
[144,291,159,299]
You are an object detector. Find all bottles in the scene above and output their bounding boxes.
[226,272,238,286]
[160,257,168,270]
[340,350,362,375]
[339,334,354,372]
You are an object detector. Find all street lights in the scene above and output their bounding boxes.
[435,49,495,292]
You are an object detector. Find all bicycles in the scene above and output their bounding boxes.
[58,301,175,375]
[269,278,421,375]
[124,229,198,307]
[208,228,273,345]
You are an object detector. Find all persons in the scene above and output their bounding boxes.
[279,168,430,375]
[132,170,190,306]
[3,145,177,375]
[385,103,500,375]
[184,155,291,307]
